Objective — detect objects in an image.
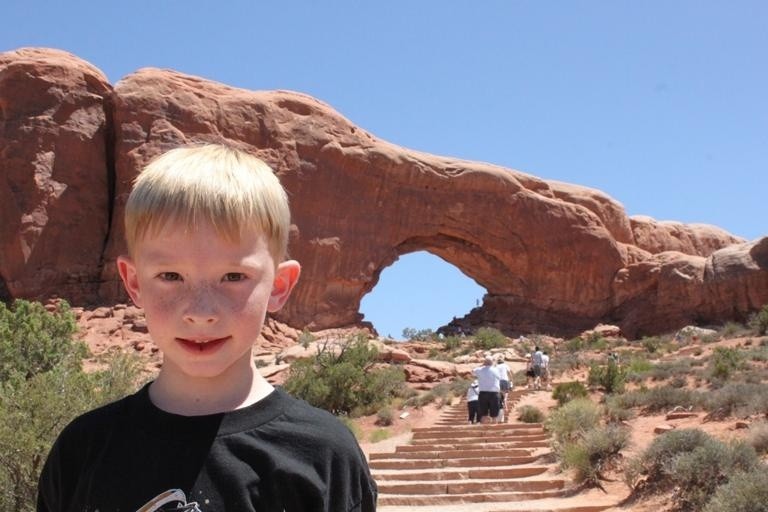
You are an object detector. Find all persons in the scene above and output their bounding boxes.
[37,143,378,511]
[526,346,552,390]
[467,354,515,424]
[438,317,475,339]
